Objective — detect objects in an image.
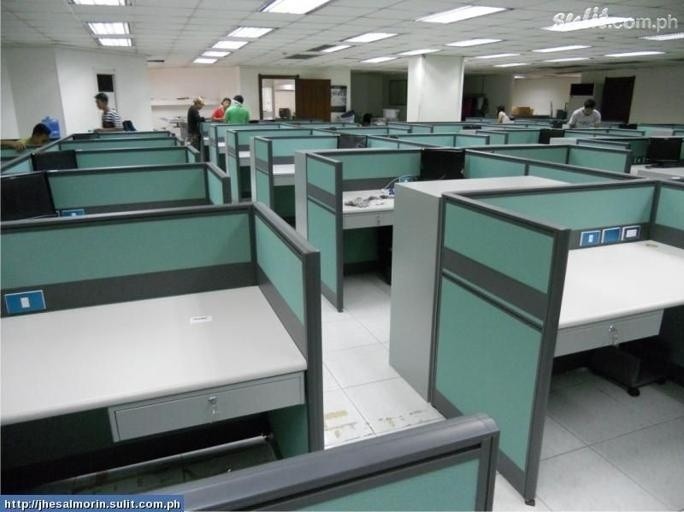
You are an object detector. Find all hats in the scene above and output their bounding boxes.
[233,95,243,105]
[193,96,207,106]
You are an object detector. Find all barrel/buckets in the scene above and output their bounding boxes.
[382,108,400,119]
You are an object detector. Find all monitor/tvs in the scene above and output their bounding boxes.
[645,138,682,168]
[73,132,99,140]
[463,125,481,130]
[337,134,367,148]
[551,121,566,128]
[539,129,565,144]
[0,171,58,222]
[31,149,77,171]
[619,124,637,129]
[420,149,465,179]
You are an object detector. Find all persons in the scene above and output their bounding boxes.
[495,104,510,124]
[93,93,124,132]
[357,113,376,127]
[187,96,205,151]
[211,97,230,122]
[567,99,601,130]
[223,95,249,124]
[0,123,50,152]
